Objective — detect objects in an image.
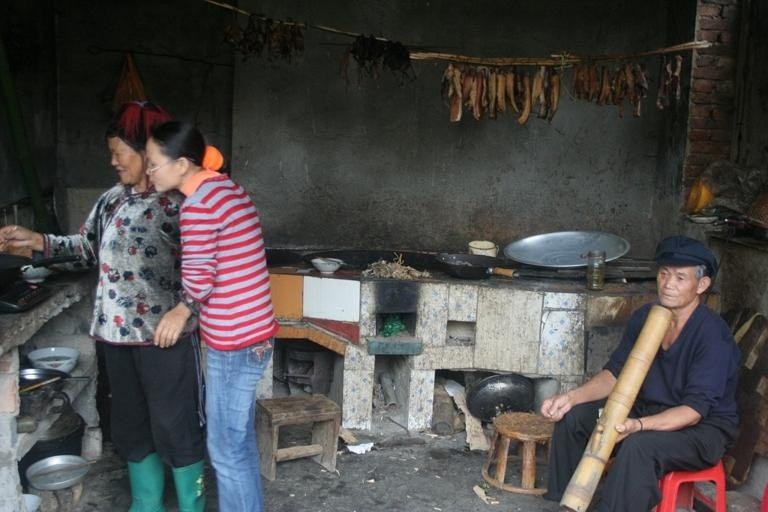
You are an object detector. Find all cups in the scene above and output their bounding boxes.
[468,240,499,258]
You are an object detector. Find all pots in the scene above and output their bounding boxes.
[433,253,520,280]
[18,367,93,385]
[0,253,84,279]
[19,414,89,482]
[465,375,538,421]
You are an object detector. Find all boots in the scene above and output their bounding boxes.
[123,451,169,512]
[169,460,207,512]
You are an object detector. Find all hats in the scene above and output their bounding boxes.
[655,236,718,277]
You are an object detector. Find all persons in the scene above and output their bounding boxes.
[1,98,207,512]
[145,117,280,512]
[540,234,742,512]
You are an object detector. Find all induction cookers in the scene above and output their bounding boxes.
[0,280,54,313]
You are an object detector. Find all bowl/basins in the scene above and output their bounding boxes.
[310,257,344,275]
[27,347,81,374]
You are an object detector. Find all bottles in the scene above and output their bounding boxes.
[585,249,607,290]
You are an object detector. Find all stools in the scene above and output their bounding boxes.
[652,459,727,512]
[482,412,557,497]
[254,392,343,482]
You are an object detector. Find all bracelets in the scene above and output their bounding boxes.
[636,417,643,431]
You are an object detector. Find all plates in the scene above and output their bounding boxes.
[686,213,717,224]
